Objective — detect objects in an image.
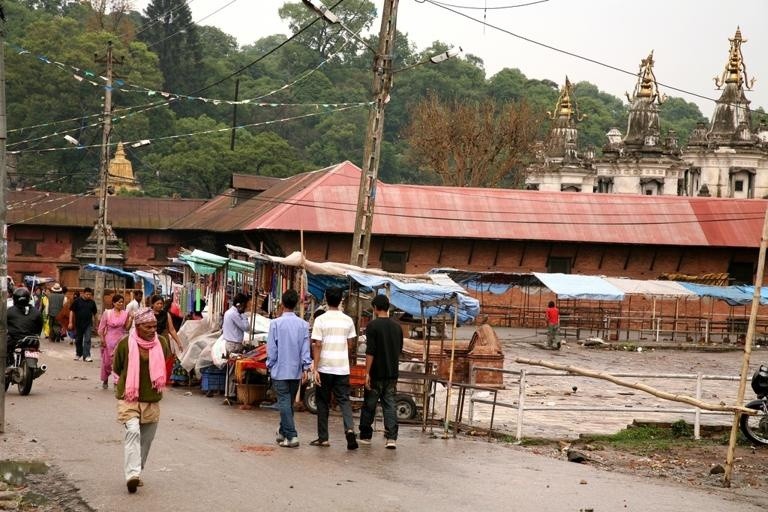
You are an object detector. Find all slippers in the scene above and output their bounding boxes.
[345,429,359,450]
[309,439,330,447]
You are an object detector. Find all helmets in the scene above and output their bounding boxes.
[12,286,30,306]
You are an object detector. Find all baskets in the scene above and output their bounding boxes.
[235,382,266,403]
[200,364,225,391]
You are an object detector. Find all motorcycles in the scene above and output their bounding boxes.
[6,332,47,394]
[739,367,767,446]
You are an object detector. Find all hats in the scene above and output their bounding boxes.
[133,307,157,324]
[50,283,63,292]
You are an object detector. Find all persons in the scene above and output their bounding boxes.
[545,301,562,350]
[97,289,206,389]
[222,294,251,404]
[266,287,403,451]
[112,306,169,496]
[5,284,97,367]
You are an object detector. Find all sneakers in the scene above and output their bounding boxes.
[73,355,80,361]
[384,439,397,448]
[279,436,299,447]
[355,438,372,445]
[83,356,93,362]
[275,425,285,442]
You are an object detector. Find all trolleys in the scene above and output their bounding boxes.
[301,349,434,419]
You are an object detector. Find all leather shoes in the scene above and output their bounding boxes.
[126,476,139,493]
[138,479,143,487]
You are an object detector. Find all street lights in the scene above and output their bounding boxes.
[64,133,152,330]
[301,0,465,328]
